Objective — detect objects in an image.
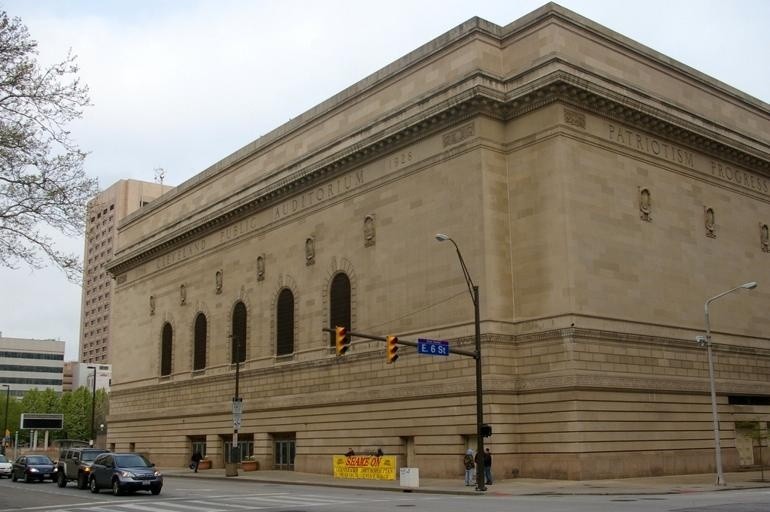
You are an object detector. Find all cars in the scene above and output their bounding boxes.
[0,437,163,495]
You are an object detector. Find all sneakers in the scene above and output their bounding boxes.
[465,483,476,486]
[485,481,493,485]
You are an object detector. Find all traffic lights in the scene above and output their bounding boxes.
[335,326,348,356]
[387,335,398,364]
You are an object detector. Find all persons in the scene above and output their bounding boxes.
[192,451,203,473]
[463,447,493,486]
[345,446,357,456]
[374,447,384,457]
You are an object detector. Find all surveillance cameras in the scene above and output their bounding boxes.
[696,335,705,343]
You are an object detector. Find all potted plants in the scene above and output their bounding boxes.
[198,456,209,469]
[239,455,257,471]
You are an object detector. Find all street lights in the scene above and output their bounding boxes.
[704,281,759,485]
[87,366,97,448]
[228,334,241,476]
[434,233,488,491]
[3,384,10,437]
